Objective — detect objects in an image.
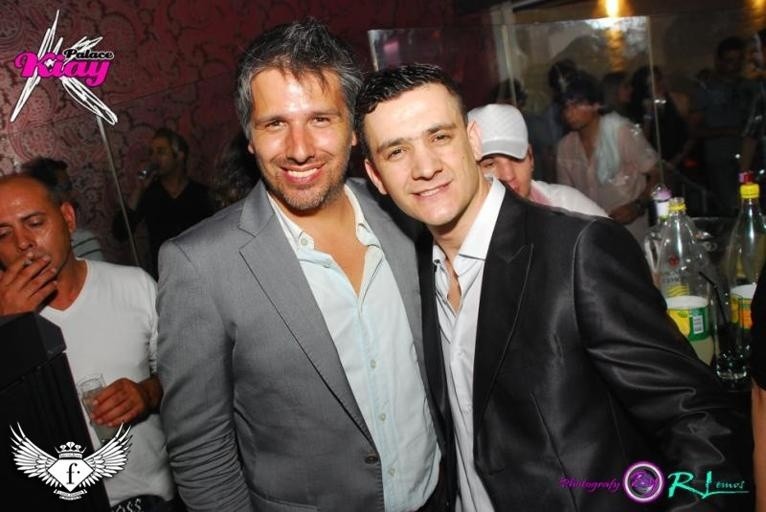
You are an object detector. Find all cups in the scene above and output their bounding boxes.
[77,373,124,449]
[707,291,748,379]
[136,163,160,181]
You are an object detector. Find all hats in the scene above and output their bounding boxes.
[467,104,529,160]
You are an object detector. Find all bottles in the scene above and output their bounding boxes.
[723,182,765,345]
[649,197,720,364]
[645,182,719,268]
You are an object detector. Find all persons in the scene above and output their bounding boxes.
[355,64,754,511]
[0,173,175,512]
[21,156,107,262]
[749,260,766,511]
[110,125,224,268]
[469,36,766,252]
[156,22,457,511]
